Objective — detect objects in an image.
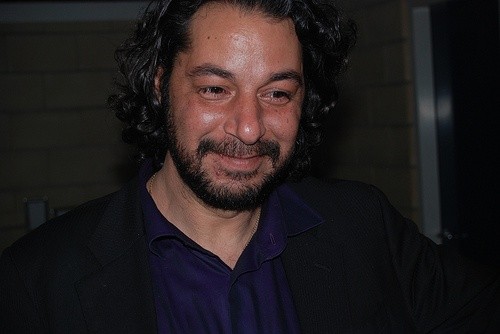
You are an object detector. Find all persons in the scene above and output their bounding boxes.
[0,0,500,334]
